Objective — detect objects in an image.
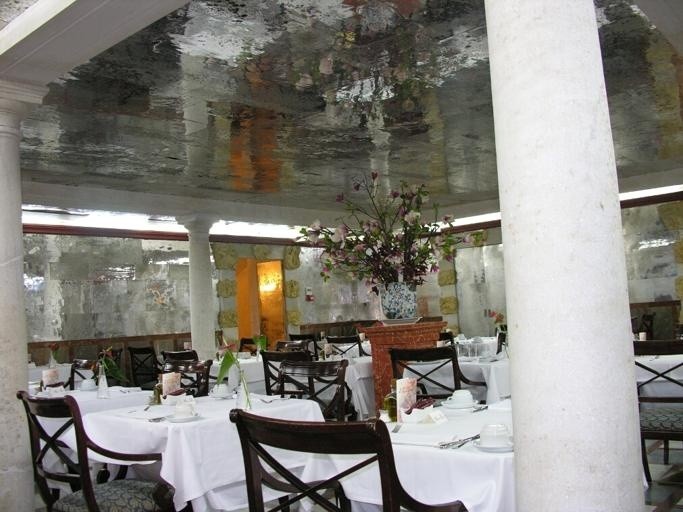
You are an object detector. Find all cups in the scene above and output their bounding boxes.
[439,390,512,453]
[455,333,507,361]
[75,378,234,423]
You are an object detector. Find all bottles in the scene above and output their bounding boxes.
[384,378,397,424]
[319,329,328,362]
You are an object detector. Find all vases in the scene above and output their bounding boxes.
[380,282,416,318]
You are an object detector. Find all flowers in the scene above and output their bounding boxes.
[295,171,474,294]
